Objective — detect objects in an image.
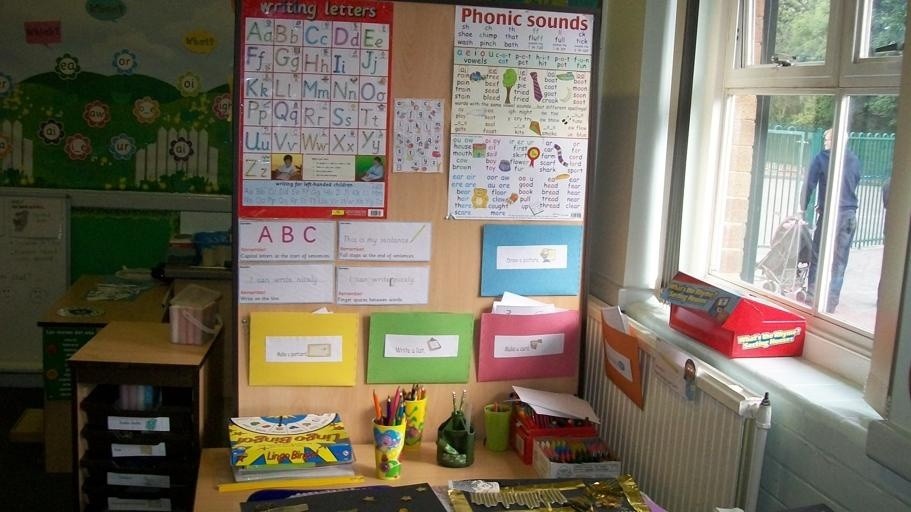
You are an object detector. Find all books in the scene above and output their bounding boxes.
[226,411,355,483]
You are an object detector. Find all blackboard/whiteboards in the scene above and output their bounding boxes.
[0,194,71,373]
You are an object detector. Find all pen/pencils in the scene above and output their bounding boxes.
[494,401,500,413]
[161,287,171,308]
[515,400,612,463]
[373,385,404,426]
[402,383,426,401]
[452,392,458,414]
[459,389,467,414]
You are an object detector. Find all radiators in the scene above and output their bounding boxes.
[581,290,779,512]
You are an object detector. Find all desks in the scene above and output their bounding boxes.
[188,447,659,511]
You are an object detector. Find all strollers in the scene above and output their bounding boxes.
[754,216,818,304]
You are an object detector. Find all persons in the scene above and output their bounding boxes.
[801,124,863,317]
[272,154,296,179]
[360,155,384,181]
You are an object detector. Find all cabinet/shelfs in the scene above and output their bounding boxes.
[153,252,239,394]
[37,261,164,474]
[55,310,224,512]
